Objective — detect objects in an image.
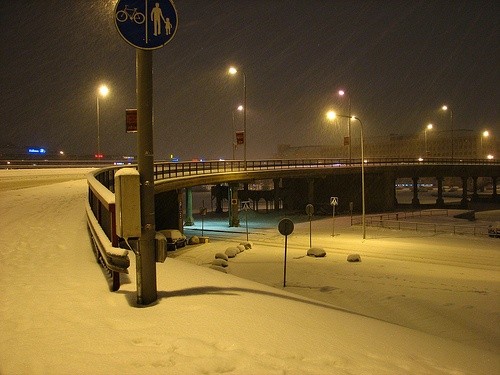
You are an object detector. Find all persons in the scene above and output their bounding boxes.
[488,224,496,238]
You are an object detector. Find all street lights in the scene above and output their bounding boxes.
[481,129,489,152]
[424,123,433,157]
[229,67,247,172]
[442,105,454,164]
[327,112,366,239]
[338,90,352,166]
[96,85,108,166]
[232,103,243,160]
